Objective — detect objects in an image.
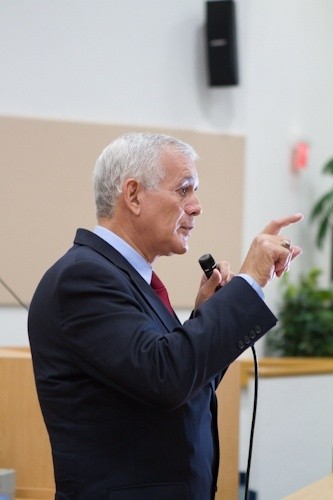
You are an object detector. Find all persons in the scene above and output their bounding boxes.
[27,130,302,500]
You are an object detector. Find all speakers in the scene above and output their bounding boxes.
[206,0,240,88]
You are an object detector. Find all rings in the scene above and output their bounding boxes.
[281,238,292,250]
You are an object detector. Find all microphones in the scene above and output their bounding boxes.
[199,255,223,293]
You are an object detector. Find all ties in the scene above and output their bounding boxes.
[151,272,173,317]
